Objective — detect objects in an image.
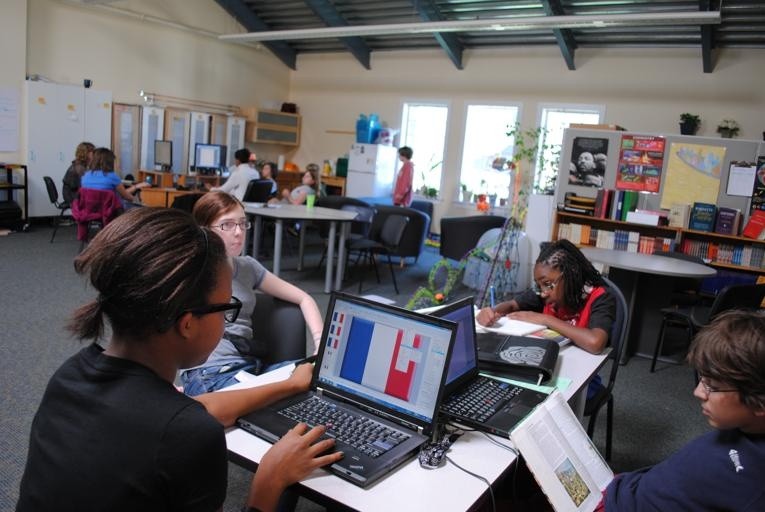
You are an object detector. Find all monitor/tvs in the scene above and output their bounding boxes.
[194,143,223,169]
[152,139,174,172]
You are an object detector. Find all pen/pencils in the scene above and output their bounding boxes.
[490,285,495,309]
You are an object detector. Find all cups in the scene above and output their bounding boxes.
[306,194,315,208]
[146,175,152,185]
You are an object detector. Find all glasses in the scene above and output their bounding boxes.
[532,272,563,296]
[211,220,251,231]
[159,295,242,334]
[697,370,739,393]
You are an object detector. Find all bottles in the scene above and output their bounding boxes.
[322,159,331,176]
[277,154,285,170]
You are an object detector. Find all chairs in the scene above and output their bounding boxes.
[584,275,629,466]
[651,283,765,385]
[643,251,706,322]
[43,172,513,296]
[256,294,305,370]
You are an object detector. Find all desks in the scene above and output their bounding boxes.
[224,303,614,512]
[579,246,718,367]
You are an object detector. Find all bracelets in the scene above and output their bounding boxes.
[593,154,598,162]
[134,184,137,189]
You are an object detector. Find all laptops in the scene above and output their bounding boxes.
[235,291,460,487]
[424,295,550,443]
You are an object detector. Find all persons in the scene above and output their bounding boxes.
[158,192,324,395]
[478,239,616,400]
[63,143,97,206]
[80,148,151,210]
[205,150,320,260]
[568,151,607,187]
[18,207,343,510]
[594,311,765,511]
[394,146,413,208]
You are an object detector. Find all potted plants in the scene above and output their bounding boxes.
[679,113,702,135]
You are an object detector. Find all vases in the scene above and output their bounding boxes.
[720,127,734,138]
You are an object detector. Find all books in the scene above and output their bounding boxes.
[474,309,572,347]
[509,385,614,511]
[556,189,765,272]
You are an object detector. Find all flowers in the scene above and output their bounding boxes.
[717,118,742,136]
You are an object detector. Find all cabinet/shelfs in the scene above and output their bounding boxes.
[245,106,303,148]
[0,164,29,233]
[552,208,765,311]
